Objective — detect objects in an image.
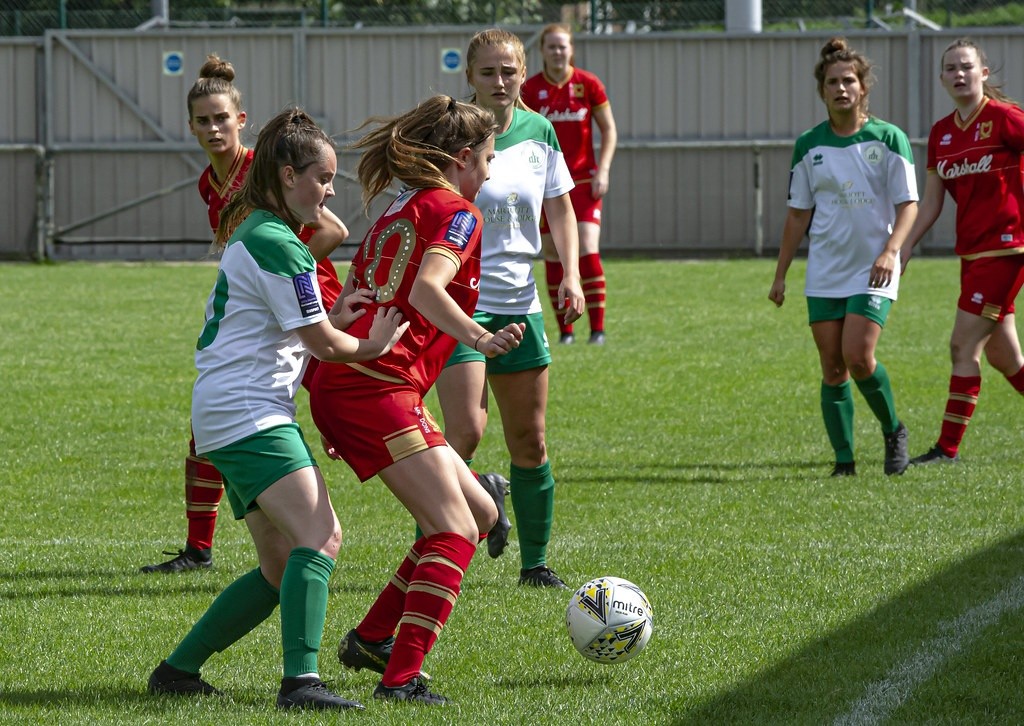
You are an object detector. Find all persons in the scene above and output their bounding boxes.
[768,34,921,479]
[897,41,1024,466]
[520,27,617,345]
[412,28,587,597]
[309,90,527,714]
[139,51,343,575]
[147,107,412,715]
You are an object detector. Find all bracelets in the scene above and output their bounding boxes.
[474,331,490,352]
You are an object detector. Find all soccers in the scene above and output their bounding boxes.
[565,576,655,664]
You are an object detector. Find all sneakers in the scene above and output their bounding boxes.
[909,442,959,465]
[276,674,362,711]
[140,541,215,573]
[560,330,606,346]
[881,421,910,475]
[144,656,222,699]
[337,627,454,707]
[482,468,576,593]
[831,462,855,476]
